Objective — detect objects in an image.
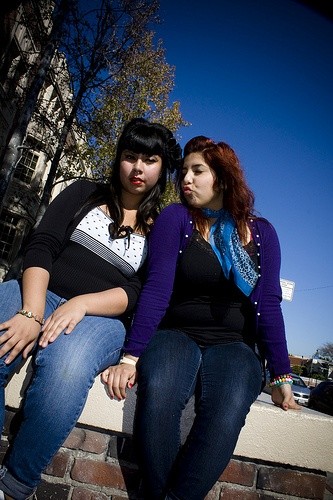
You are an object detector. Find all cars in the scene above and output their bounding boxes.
[263,369,333,417]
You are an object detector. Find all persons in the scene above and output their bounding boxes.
[101,136,303,500]
[1,118,183,500]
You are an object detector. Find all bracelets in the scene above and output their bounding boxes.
[118,356,139,367]
[266,374,295,386]
[16,309,45,326]
